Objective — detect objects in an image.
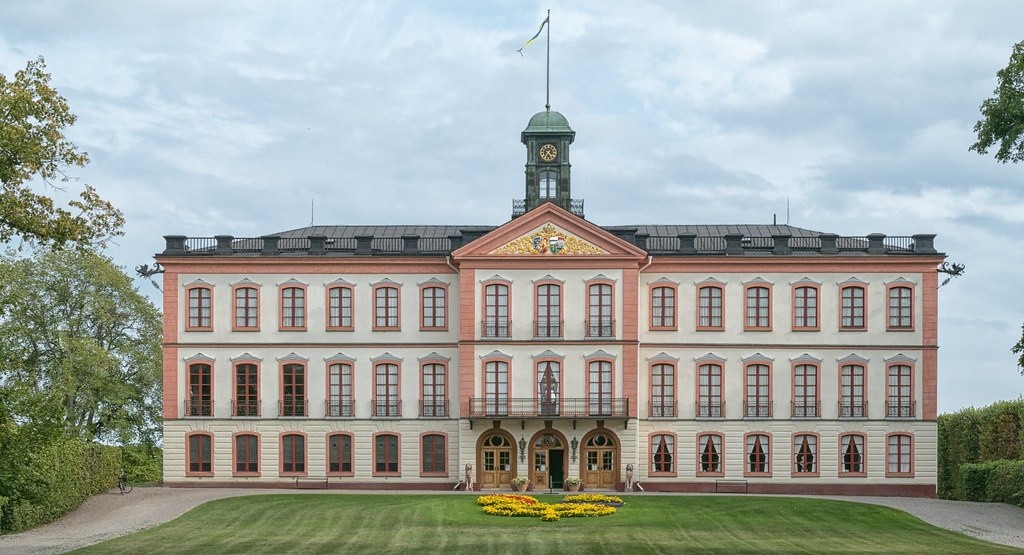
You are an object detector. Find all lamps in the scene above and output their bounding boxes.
[570,436,578,463]
[519,434,527,463]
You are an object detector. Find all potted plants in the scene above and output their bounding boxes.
[511,476,530,492]
[566,478,582,492]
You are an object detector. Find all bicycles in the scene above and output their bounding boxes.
[118,469,133,495]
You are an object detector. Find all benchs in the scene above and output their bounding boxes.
[716,479,748,493]
[296,476,328,490]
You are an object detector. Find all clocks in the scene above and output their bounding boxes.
[538,142,558,163]
